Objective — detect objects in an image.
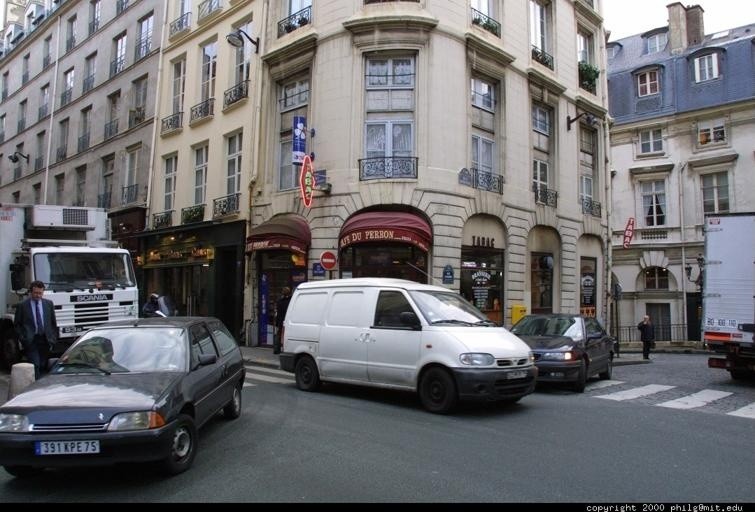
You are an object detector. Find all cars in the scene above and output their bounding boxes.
[508,312,615,393]
[0,317,244,478]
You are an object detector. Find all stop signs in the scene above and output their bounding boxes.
[320,250,336,271]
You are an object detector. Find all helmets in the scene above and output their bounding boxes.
[151,293,158,299]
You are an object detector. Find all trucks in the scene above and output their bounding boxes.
[701,210,755,384]
[0,203,140,375]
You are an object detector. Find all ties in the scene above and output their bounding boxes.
[36,302,43,335]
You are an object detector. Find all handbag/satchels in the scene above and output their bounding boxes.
[651,342,656,348]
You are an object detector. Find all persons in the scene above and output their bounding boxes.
[142,294,165,317]
[13,279,59,380]
[637,313,656,362]
[274,286,294,358]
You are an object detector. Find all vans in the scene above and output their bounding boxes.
[279,277,539,414]
